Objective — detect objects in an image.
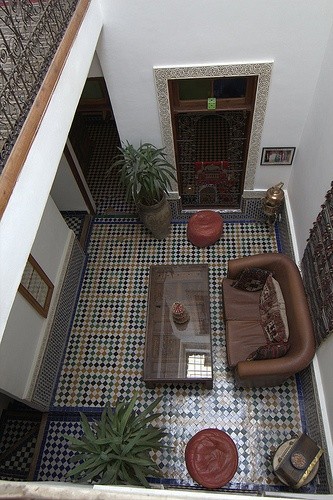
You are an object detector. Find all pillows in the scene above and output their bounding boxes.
[246,344,290,361]
[259,273,289,344]
[230,267,272,292]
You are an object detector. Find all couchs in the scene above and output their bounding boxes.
[222,253,315,387]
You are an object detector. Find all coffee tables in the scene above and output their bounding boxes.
[143,263,214,390]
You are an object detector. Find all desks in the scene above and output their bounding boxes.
[273,438,319,488]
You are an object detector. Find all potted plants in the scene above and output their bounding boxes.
[114,138,178,240]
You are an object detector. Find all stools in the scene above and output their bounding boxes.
[187,210,224,248]
[185,428,238,489]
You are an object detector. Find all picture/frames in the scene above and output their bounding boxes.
[260,147,296,166]
[17,253,55,319]
